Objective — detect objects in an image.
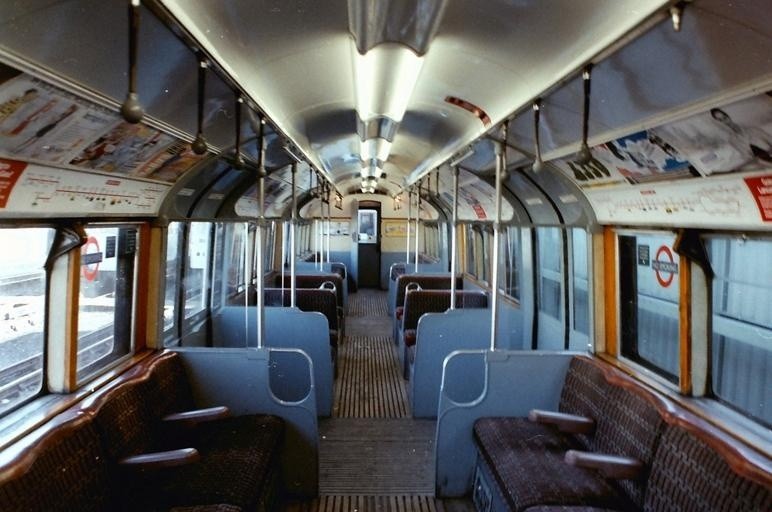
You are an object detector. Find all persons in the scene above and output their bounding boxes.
[710,104,772,171]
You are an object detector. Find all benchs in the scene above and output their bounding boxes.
[5,351,317,508]
[450,352,772,510]
[174,255,346,417]
[391,258,523,417]
